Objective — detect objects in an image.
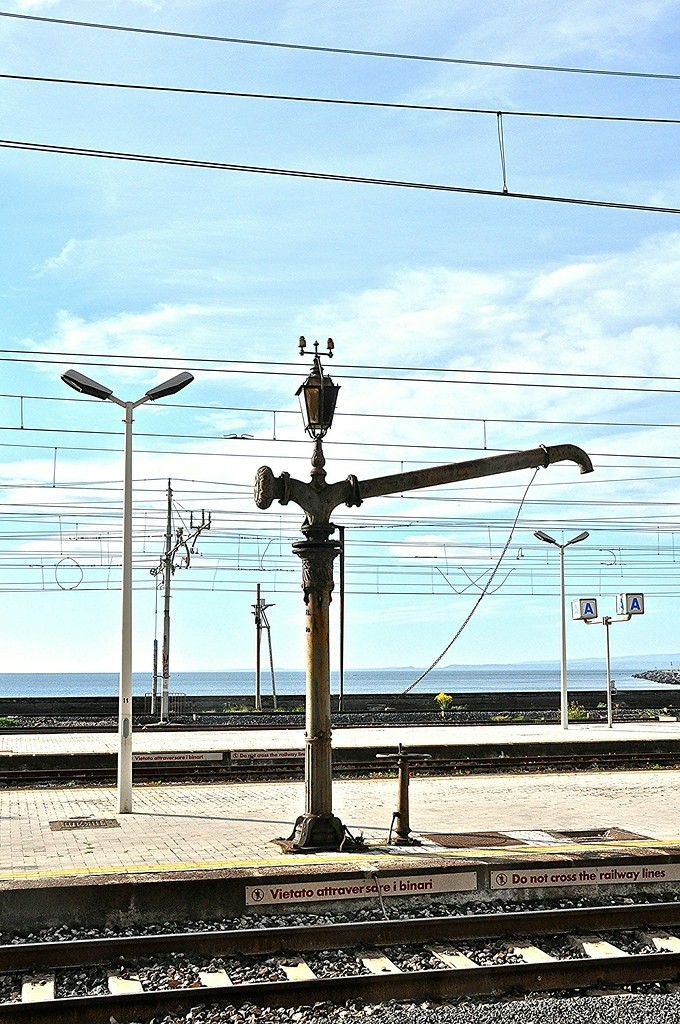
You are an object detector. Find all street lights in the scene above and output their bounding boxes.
[60,366,197,815]
[280,333,360,851]
[533,528,590,731]
